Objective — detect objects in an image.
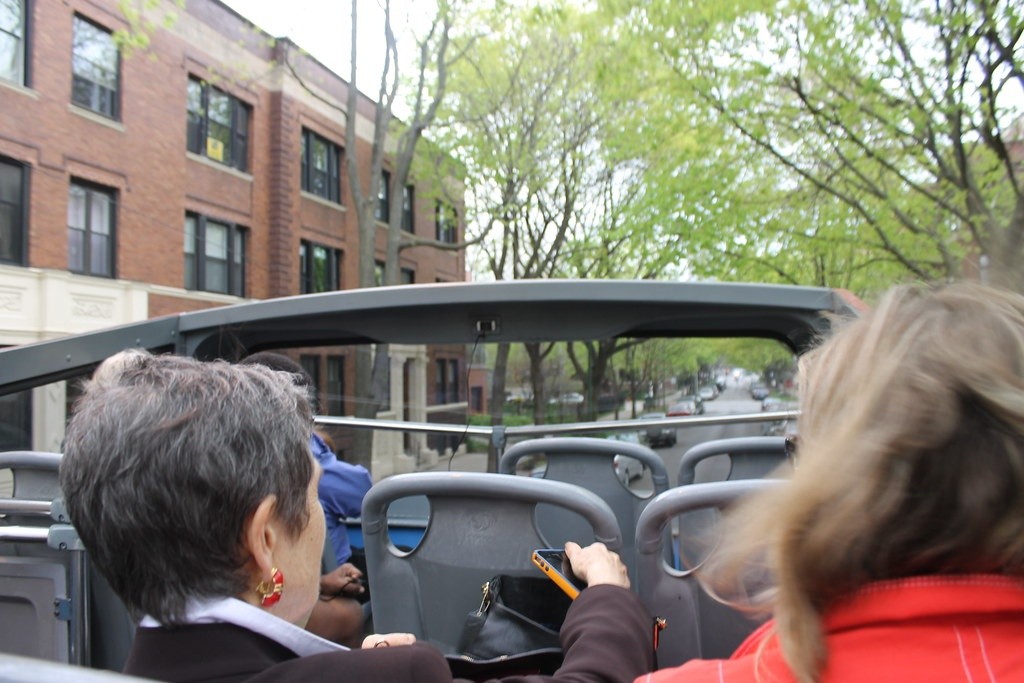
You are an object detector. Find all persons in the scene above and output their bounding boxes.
[239,351,415,580]
[632,279,1024,683]
[59,349,656,683]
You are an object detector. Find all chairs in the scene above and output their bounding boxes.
[0,430,807,683]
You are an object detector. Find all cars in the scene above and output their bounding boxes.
[635,413,677,448]
[699,384,719,399]
[550,393,584,403]
[759,396,799,436]
[750,384,769,400]
[665,396,706,417]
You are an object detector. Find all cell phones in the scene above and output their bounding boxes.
[532,549,588,600]
